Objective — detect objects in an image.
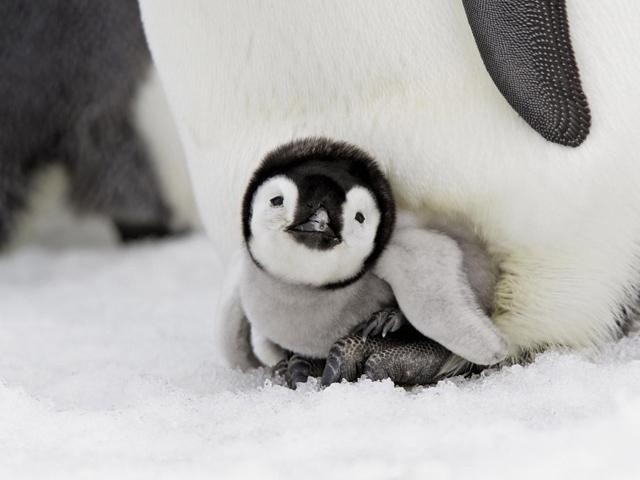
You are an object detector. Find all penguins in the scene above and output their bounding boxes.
[216,137,508,379]
[136,1,640,388]
[0,1,199,253]
